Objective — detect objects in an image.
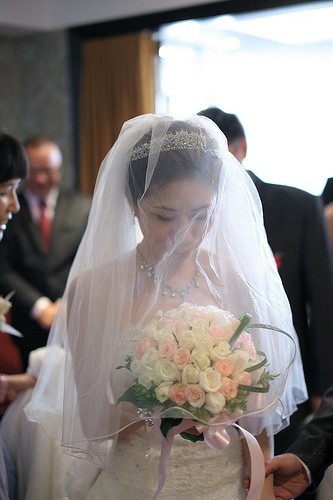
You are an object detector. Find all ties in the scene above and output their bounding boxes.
[36,197,53,252]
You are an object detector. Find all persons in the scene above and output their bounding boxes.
[196,107,333,456]
[245,387,333,500]
[67,122,274,500]
[0,134,91,373]
[0,132,38,403]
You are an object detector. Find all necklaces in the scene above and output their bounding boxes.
[136,242,201,298]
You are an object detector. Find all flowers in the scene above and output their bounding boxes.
[0,296,24,337]
[114,308,282,444]
